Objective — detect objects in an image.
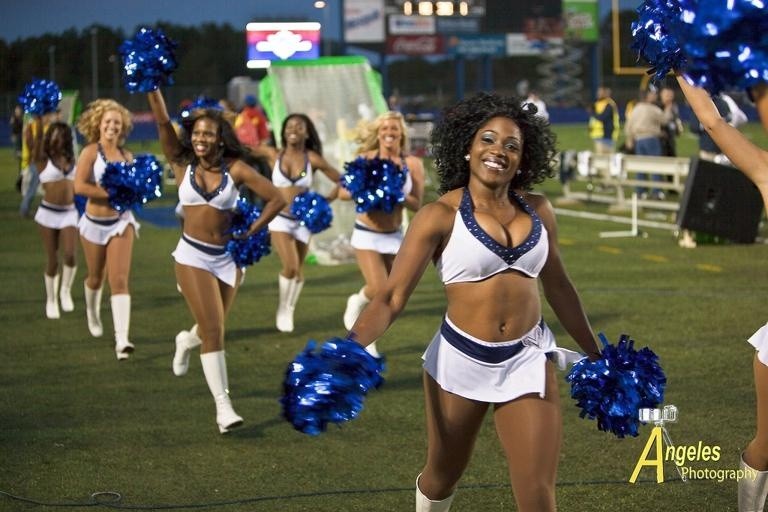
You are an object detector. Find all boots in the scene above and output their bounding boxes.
[276,273,303,332]
[83,279,103,337]
[44,265,77,319]
[172,324,202,376]
[200,350,244,433]
[344,288,377,357]
[110,294,134,360]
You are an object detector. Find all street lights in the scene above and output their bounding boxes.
[109,52,122,102]
[46,44,56,85]
[89,25,101,102]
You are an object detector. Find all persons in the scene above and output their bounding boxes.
[74,99,140,360]
[521,90,547,118]
[232,96,269,146]
[587,84,748,201]
[337,111,425,358]
[346,91,601,512]
[673,68,768,512]
[9,105,79,320]
[146,85,285,434]
[249,114,342,334]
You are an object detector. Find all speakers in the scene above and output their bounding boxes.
[673,155,766,244]
